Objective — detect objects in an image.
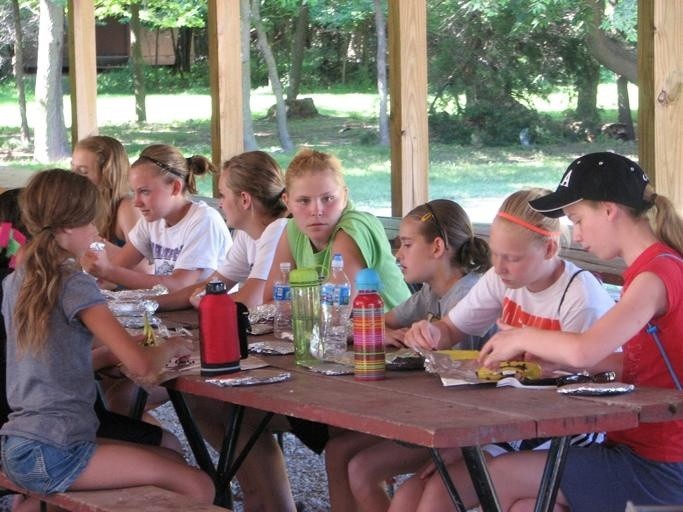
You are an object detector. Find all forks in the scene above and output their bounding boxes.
[157,323,194,340]
[496,377,557,391]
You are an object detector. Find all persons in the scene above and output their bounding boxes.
[1,136,683,512]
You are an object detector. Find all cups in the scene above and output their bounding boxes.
[288,266,334,365]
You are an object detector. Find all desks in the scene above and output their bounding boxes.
[469,222,630,287]
[94,309,682,512]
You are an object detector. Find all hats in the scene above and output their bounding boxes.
[527,152,652,218]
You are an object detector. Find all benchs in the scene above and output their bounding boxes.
[0,463,236,511]
[374,215,423,295]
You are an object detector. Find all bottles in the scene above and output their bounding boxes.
[272,262,293,340]
[350,267,386,381]
[196,276,251,376]
[318,253,352,356]
